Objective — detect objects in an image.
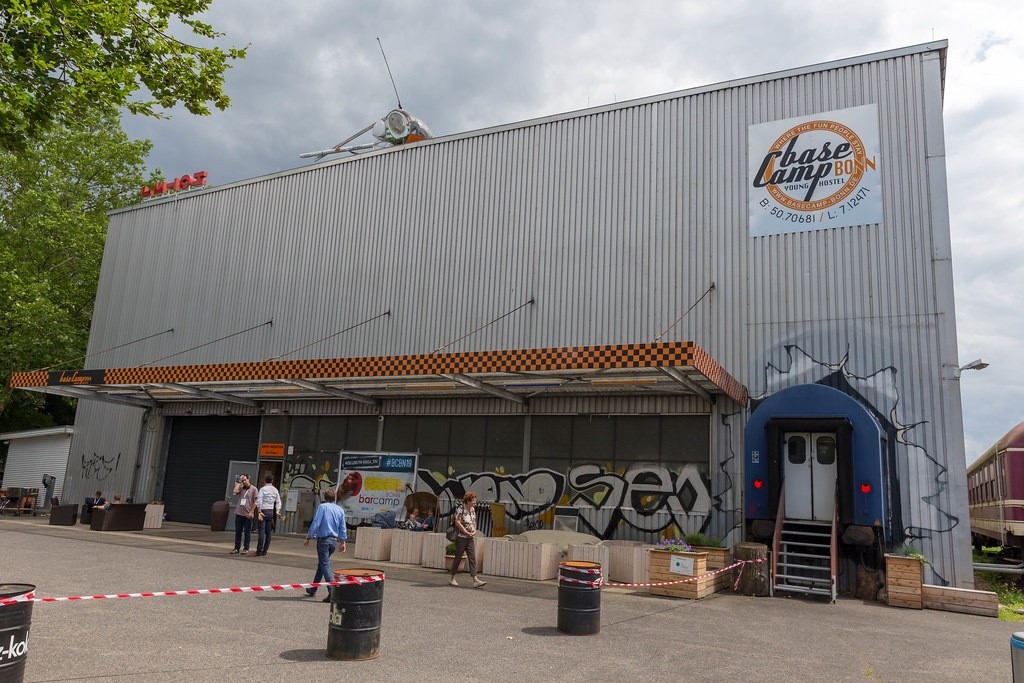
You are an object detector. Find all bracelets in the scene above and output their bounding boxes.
[258,512,261,513]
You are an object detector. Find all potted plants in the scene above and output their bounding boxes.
[884,543,930,610]
[682,531,732,595]
[444,542,467,572]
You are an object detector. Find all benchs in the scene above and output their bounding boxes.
[421,527,655,588]
[90,502,148,531]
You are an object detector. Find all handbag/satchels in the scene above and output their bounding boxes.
[446,525,459,542]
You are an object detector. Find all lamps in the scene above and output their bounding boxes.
[953,359,989,378]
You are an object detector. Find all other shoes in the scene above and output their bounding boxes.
[449,579,458,586]
[241,550,248,555]
[306,587,317,597]
[230,549,238,554]
[474,581,487,588]
[323,594,330,603]
[256,551,267,556]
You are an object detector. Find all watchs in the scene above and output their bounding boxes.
[238,480,242,483]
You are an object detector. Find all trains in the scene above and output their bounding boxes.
[967,422,1024,556]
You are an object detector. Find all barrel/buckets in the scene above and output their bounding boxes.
[326,568,384,662]
[0,582,35,683]
[557,560,603,636]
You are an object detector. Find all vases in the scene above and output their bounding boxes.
[649,548,709,599]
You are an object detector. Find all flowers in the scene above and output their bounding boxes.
[655,539,693,553]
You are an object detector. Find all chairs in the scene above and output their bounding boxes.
[144,504,164,528]
[80,497,105,524]
[49,497,79,526]
[354,527,434,565]
[568,544,609,583]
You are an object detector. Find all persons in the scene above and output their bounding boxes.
[303,488,347,602]
[448,491,487,588]
[405,508,434,531]
[229,473,282,556]
[88,491,134,513]
